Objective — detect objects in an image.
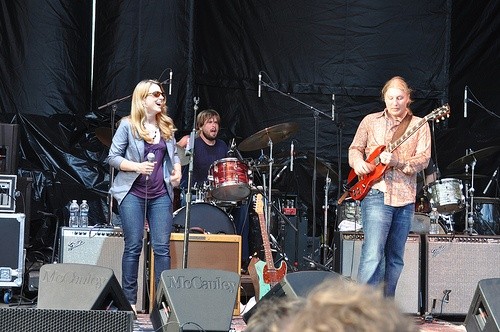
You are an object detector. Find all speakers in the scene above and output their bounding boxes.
[243,232,500,332]
[0,123,20,175]
[0,226,148,332]
[149,232,243,332]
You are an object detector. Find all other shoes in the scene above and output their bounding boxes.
[130,304,138,316]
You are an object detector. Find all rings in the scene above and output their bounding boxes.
[384,158,387,161]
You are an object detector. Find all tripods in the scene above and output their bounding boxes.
[307,171,334,267]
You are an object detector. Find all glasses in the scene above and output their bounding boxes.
[143,91,167,98]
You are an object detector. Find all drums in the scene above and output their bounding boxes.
[426,178,466,213]
[208,157,251,202]
[172,201,237,235]
[467,196,499,236]
[408,212,450,235]
[337,201,364,232]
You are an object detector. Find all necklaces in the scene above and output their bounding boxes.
[152,128,158,139]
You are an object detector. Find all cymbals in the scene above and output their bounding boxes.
[238,122,302,152]
[176,144,196,166]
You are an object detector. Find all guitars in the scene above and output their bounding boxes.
[342,102,450,202]
[247,194,288,305]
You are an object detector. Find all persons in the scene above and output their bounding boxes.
[108,80,183,315]
[348,75,431,300]
[178,109,228,204]
[239,277,423,332]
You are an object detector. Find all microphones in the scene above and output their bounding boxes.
[464,87,468,117]
[258,72,262,97]
[290,141,294,171]
[227,138,235,157]
[274,166,287,182]
[145,153,155,180]
[169,70,173,95]
[332,93,334,120]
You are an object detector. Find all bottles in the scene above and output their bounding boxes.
[68,200,80,227]
[79,199,89,227]
[429,208,439,234]
